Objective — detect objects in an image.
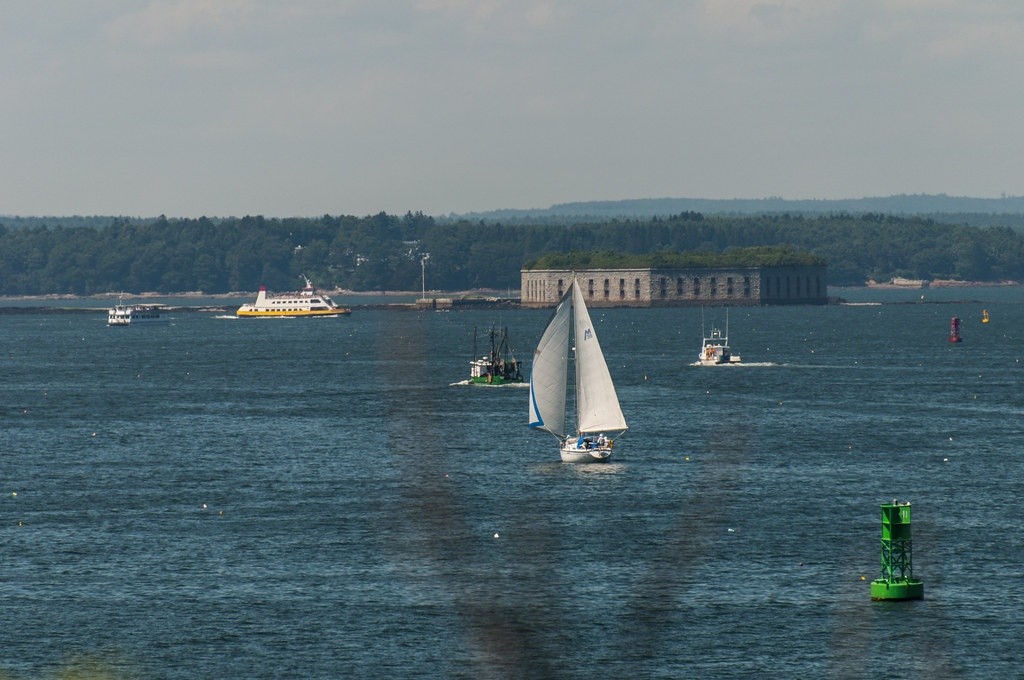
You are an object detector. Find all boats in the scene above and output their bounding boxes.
[236,279,351,316]
[108,290,170,327]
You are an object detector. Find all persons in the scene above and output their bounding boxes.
[597,434,604,446]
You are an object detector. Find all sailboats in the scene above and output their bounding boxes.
[688,303,742,366]
[526,271,629,462]
[448,314,529,386]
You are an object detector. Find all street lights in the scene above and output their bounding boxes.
[420,254,429,298]
